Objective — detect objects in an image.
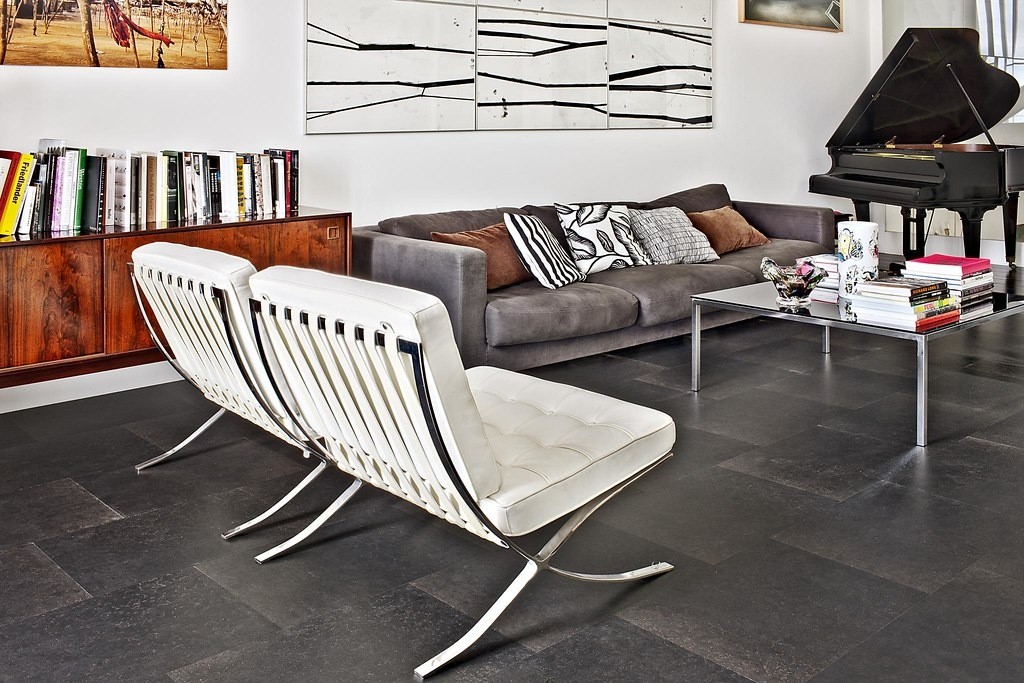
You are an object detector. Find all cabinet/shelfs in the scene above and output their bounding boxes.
[0,207,353,389]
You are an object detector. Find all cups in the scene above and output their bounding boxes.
[836,221,879,299]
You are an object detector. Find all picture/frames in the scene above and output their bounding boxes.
[737,0,843,33]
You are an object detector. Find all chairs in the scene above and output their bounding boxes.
[127,239,339,540]
[247,266,676,677]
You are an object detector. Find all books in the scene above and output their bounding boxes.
[0,146,300,235]
[797,254,995,330]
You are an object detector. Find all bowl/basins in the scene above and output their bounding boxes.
[759,257,828,305]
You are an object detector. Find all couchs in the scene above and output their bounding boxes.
[353,202,835,369]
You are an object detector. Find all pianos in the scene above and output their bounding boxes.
[806,27,1024,264]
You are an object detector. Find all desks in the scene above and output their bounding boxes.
[689,279,1024,447]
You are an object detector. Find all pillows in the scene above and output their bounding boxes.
[378,207,528,240]
[688,204,772,254]
[627,205,721,264]
[503,212,587,290]
[554,203,655,275]
[636,184,733,213]
[522,204,573,256]
[431,222,533,290]
